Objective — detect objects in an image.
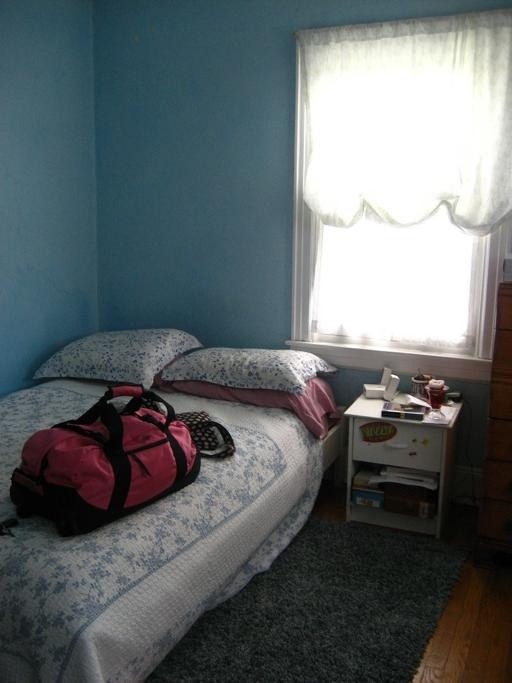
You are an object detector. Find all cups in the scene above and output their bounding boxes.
[425,386,448,420]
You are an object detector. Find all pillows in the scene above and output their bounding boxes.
[161,347,340,393]
[32,330,204,391]
[154,372,336,440]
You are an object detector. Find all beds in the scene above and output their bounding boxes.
[0,377,346,683]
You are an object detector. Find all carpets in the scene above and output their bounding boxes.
[141,515,470,683]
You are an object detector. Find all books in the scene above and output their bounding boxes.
[382,402,426,420]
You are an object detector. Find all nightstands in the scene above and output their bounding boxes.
[344,390,462,540]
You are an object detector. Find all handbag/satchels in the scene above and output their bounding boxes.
[9,385,202,537]
[172,411,218,451]
